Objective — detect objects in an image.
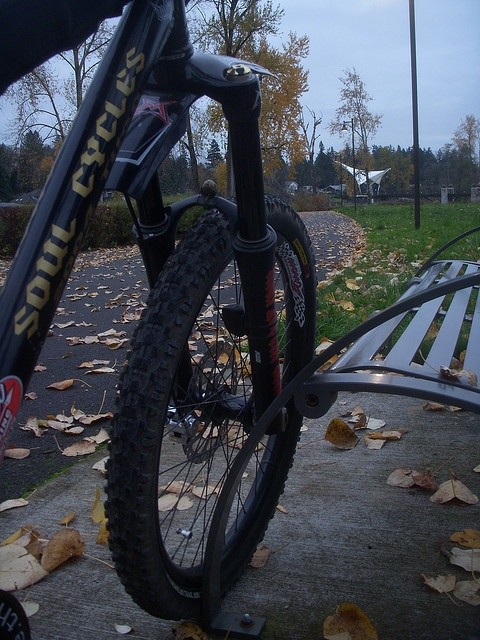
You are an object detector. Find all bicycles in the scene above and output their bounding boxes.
[0,0,318,622]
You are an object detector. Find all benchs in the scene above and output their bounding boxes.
[294,224,480,419]
